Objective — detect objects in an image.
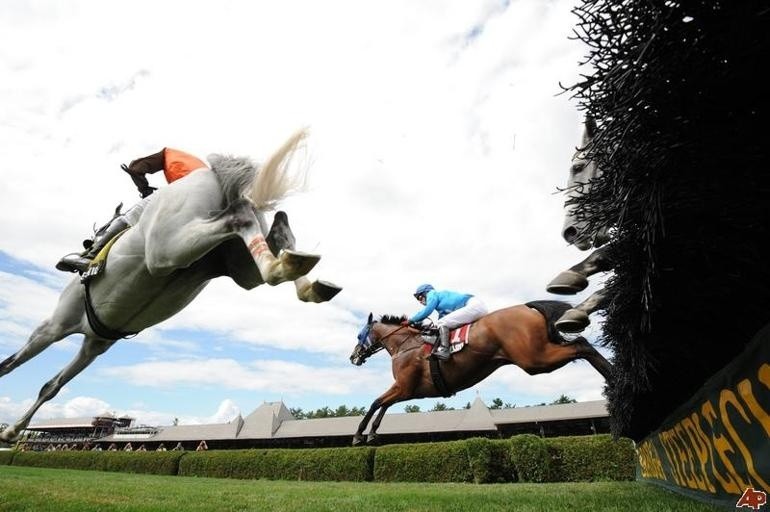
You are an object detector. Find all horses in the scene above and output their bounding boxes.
[347,298,614,447]
[545,108,627,334]
[0,126,344,447]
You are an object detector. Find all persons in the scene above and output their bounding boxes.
[21,440,133,452]
[196,440,208,451]
[55,148,210,276]
[136,445,147,452]
[172,442,184,451]
[156,443,167,452]
[401,284,487,361]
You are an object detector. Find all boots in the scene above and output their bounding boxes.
[55,219,132,272]
[425,325,451,362]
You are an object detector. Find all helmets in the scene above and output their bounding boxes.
[413,284,434,300]
